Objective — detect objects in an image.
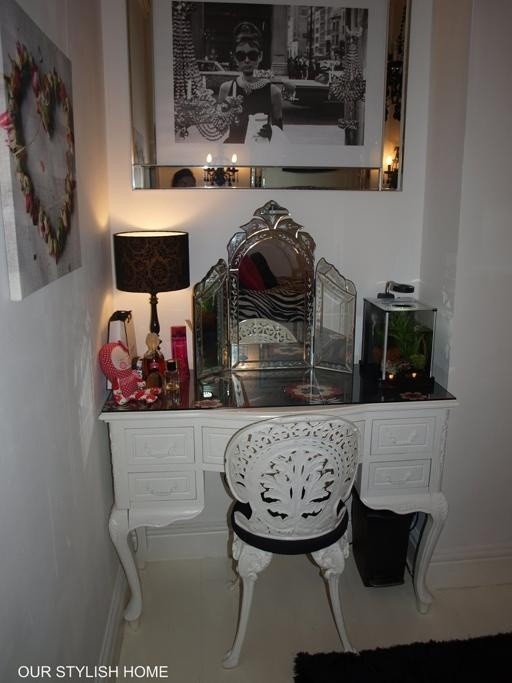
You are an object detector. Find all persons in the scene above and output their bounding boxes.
[172,167,197,188]
[217,21,285,142]
[285,55,323,81]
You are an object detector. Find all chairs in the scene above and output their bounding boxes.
[219,414,363,670]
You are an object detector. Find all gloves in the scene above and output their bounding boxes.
[257,123,273,141]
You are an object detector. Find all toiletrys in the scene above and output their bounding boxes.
[143,367,163,388]
[164,359,181,390]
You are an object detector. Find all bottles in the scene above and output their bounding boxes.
[144,326,190,399]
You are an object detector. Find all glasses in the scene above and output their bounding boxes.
[233,49,261,63]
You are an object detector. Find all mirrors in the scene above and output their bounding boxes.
[190,199,357,380]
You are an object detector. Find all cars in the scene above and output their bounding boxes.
[280,71,345,108]
[194,60,246,91]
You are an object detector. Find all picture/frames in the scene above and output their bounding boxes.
[123,0,411,190]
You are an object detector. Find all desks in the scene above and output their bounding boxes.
[94,369,459,632]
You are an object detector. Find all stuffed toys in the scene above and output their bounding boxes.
[98,339,164,406]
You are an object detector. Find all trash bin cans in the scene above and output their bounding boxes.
[351,494,416,587]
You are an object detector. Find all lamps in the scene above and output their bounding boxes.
[111,228,189,357]
